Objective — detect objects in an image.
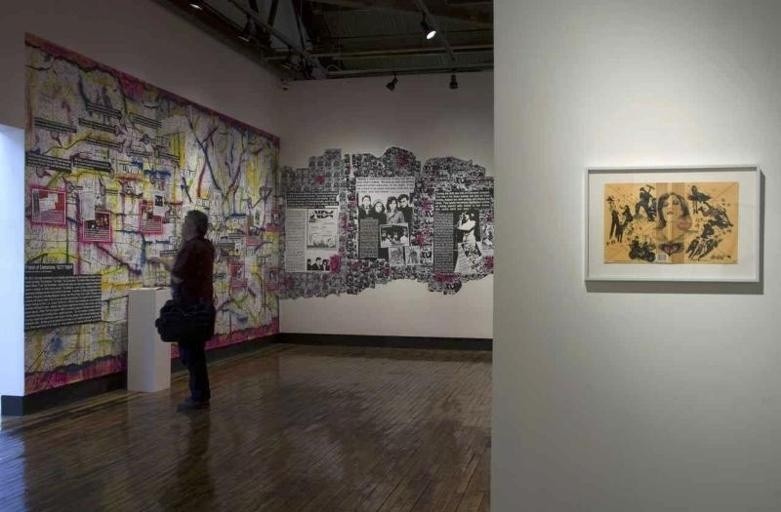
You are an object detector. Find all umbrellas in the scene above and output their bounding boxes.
[178,397,210,411]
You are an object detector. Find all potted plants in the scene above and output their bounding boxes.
[587,166,761,283]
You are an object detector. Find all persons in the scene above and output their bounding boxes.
[454,210,477,257]
[171,210,214,410]
[605,183,738,264]
[356,194,413,223]
[307,257,329,270]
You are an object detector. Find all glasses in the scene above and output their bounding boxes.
[421,11,437,39]
[238,15,255,41]
[188,0,205,11]
[386,73,399,91]
[448,73,458,89]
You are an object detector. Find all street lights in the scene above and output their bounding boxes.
[155,300,216,342]
